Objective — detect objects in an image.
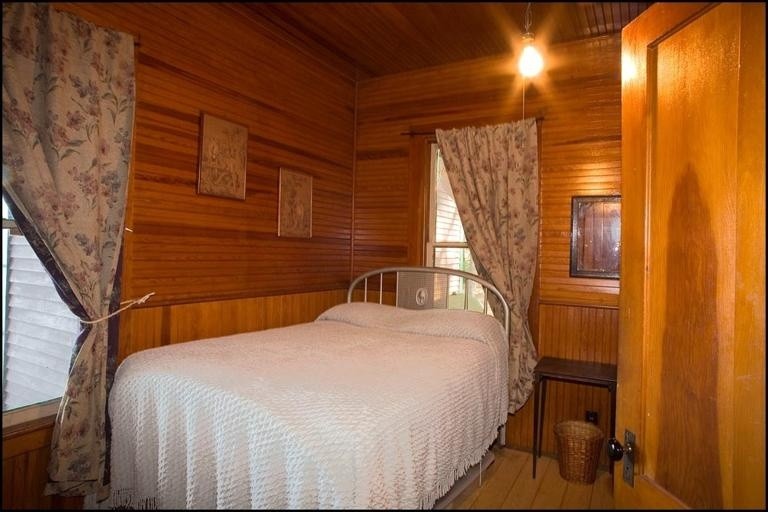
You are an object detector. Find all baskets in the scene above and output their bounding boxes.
[554,420,604,485]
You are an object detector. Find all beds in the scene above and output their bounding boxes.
[109,266,510,509]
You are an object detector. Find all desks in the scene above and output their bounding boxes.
[532,356,616,478]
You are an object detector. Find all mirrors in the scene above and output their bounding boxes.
[569,194,622,279]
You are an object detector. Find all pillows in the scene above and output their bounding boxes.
[315,301,507,345]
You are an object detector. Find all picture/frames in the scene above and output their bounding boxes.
[278,166,313,239]
[196,109,248,201]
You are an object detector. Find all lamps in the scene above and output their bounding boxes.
[520,2,543,75]
